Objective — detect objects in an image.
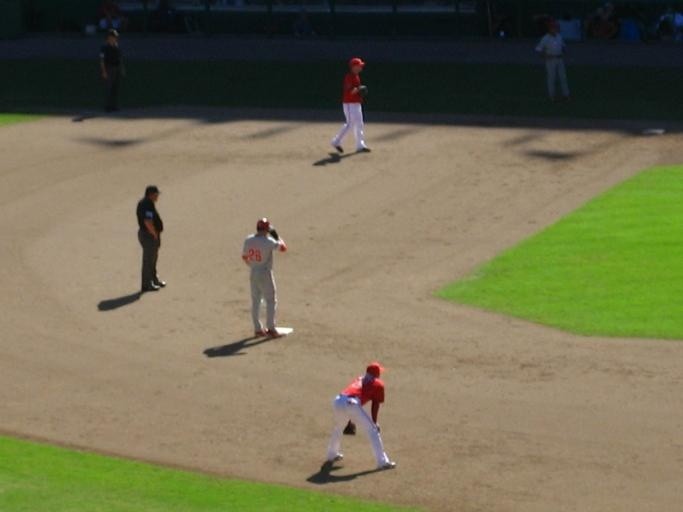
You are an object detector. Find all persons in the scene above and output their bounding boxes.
[100,30,126,112]
[242,218,288,337]
[535,20,574,103]
[330,58,371,153]
[327,363,396,469]
[136,185,166,292]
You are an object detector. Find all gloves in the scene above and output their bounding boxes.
[268,226,277,240]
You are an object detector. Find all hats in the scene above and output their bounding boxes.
[145,186,161,194]
[348,58,364,67]
[104,29,119,37]
[366,363,384,376]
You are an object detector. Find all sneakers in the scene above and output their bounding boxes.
[383,462,395,468]
[335,144,342,152]
[266,330,281,337]
[254,328,266,337]
[142,284,159,291]
[334,453,343,460]
[356,148,369,152]
[155,280,166,287]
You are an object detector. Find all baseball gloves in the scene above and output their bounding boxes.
[357,85,367,95]
[267,225,278,241]
[343,420,356,434]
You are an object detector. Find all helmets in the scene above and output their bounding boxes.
[256,218,269,230]
